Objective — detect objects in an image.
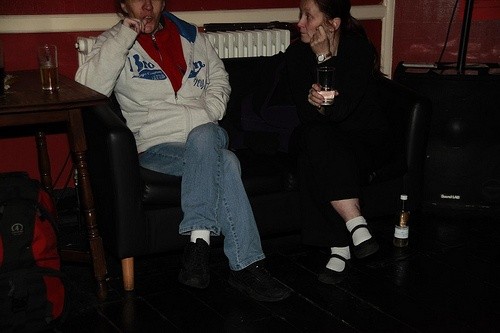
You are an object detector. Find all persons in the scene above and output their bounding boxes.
[75,0,292,304]
[264,0,393,285]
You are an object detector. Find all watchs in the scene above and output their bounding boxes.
[316,52,331,62]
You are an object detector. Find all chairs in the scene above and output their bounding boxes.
[0,197,71,333]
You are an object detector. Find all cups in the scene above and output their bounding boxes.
[38,45,60,91]
[317,67,336,105]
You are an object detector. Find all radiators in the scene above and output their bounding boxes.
[74,29,291,68]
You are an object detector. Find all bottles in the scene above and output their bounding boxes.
[392,195,410,247]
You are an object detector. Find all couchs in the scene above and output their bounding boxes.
[79,60,434,296]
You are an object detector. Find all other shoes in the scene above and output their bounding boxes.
[184,239,213,293]
[230,263,291,301]
[350,223,379,259]
[319,254,350,285]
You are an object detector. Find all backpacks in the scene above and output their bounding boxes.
[1,172,65,330]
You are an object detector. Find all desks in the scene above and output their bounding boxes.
[0,68,110,303]
